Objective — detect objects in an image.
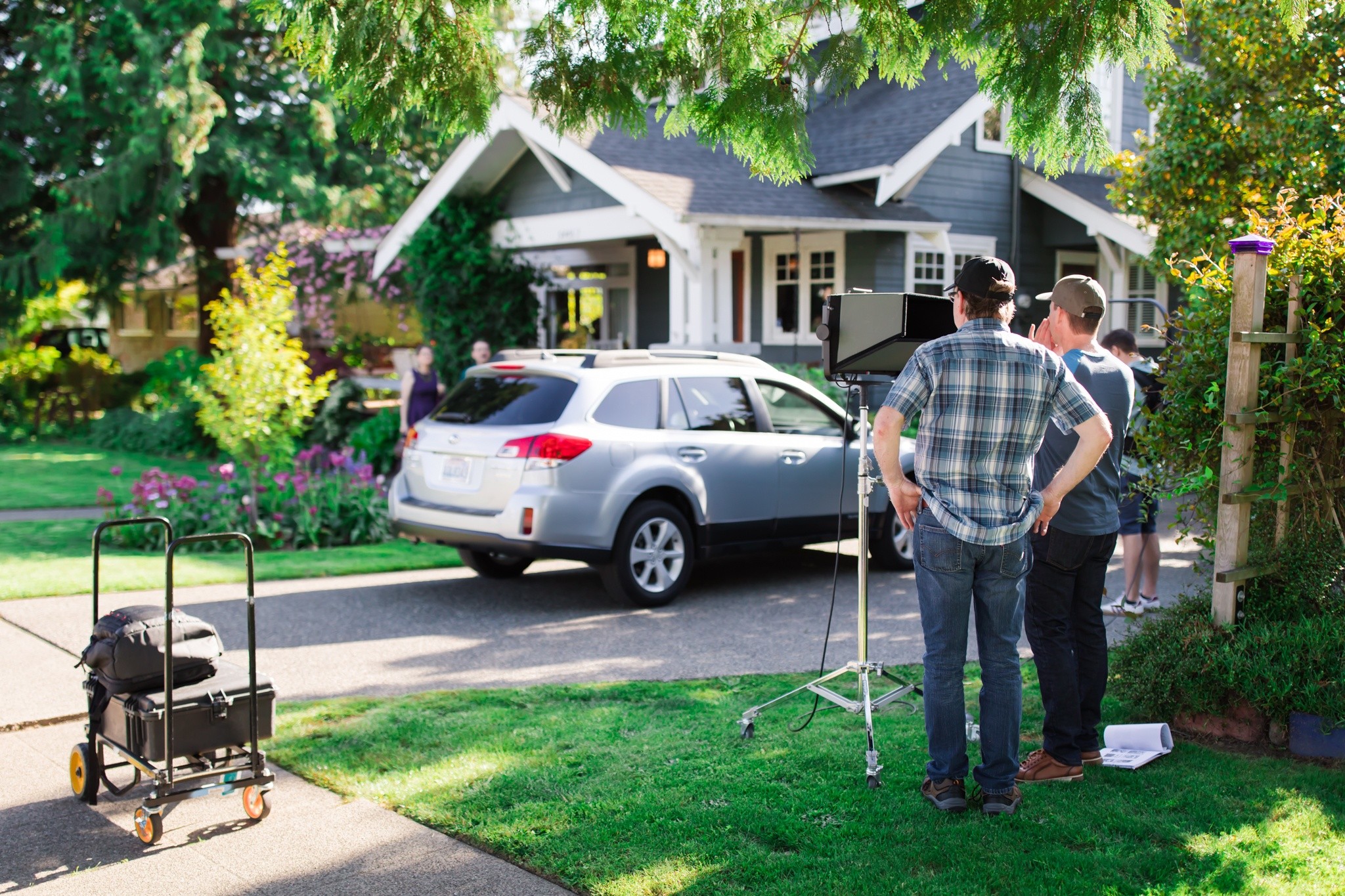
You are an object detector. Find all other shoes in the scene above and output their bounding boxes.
[1100,593,1144,617]
[1139,593,1160,611]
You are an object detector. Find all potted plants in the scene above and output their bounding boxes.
[1174,608,1345,760]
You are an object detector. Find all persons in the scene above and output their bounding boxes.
[1101,328,1166,618]
[872,256,1114,817]
[1014,274,1135,784]
[459,338,492,380]
[399,344,440,451]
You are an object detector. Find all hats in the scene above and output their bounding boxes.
[943,256,1015,301]
[1035,274,1106,318]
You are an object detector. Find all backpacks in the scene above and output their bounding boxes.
[82,603,223,697]
[1131,368,1172,432]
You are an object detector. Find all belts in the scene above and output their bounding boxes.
[922,499,929,508]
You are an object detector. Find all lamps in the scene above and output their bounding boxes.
[740,287,981,789]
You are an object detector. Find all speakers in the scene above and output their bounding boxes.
[816,292,959,372]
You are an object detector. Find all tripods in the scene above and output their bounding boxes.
[738,376,981,793]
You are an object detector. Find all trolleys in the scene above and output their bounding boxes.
[67,514,277,846]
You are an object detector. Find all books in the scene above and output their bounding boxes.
[1099,722,1174,770]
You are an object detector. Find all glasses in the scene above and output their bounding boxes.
[948,292,957,303]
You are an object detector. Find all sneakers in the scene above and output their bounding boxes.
[1081,750,1103,765]
[969,782,1021,815]
[921,775,966,809]
[1013,748,1084,783]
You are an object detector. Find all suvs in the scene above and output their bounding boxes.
[385,345,922,610]
